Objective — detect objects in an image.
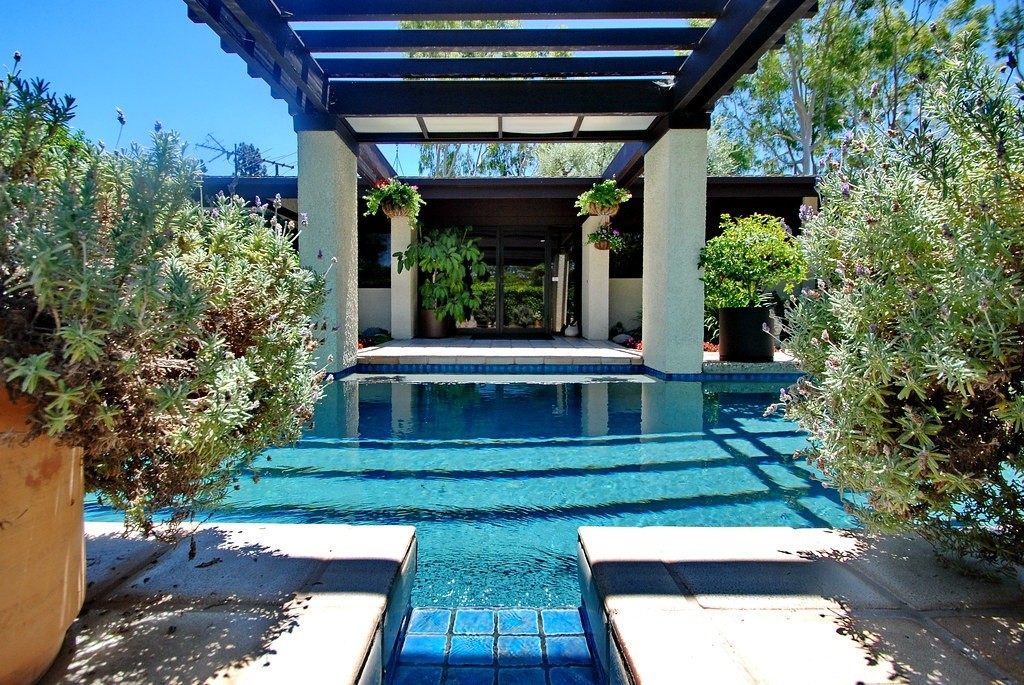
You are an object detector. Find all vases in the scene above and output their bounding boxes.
[381,195,413,218]
[594,239,611,250]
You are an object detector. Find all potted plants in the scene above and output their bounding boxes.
[0,47,332,685]
[696,211,809,364]
[392,224,490,338]
[574,178,633,217]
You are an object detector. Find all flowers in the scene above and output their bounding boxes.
[586,220,627,255]
[362,177,427,230]
